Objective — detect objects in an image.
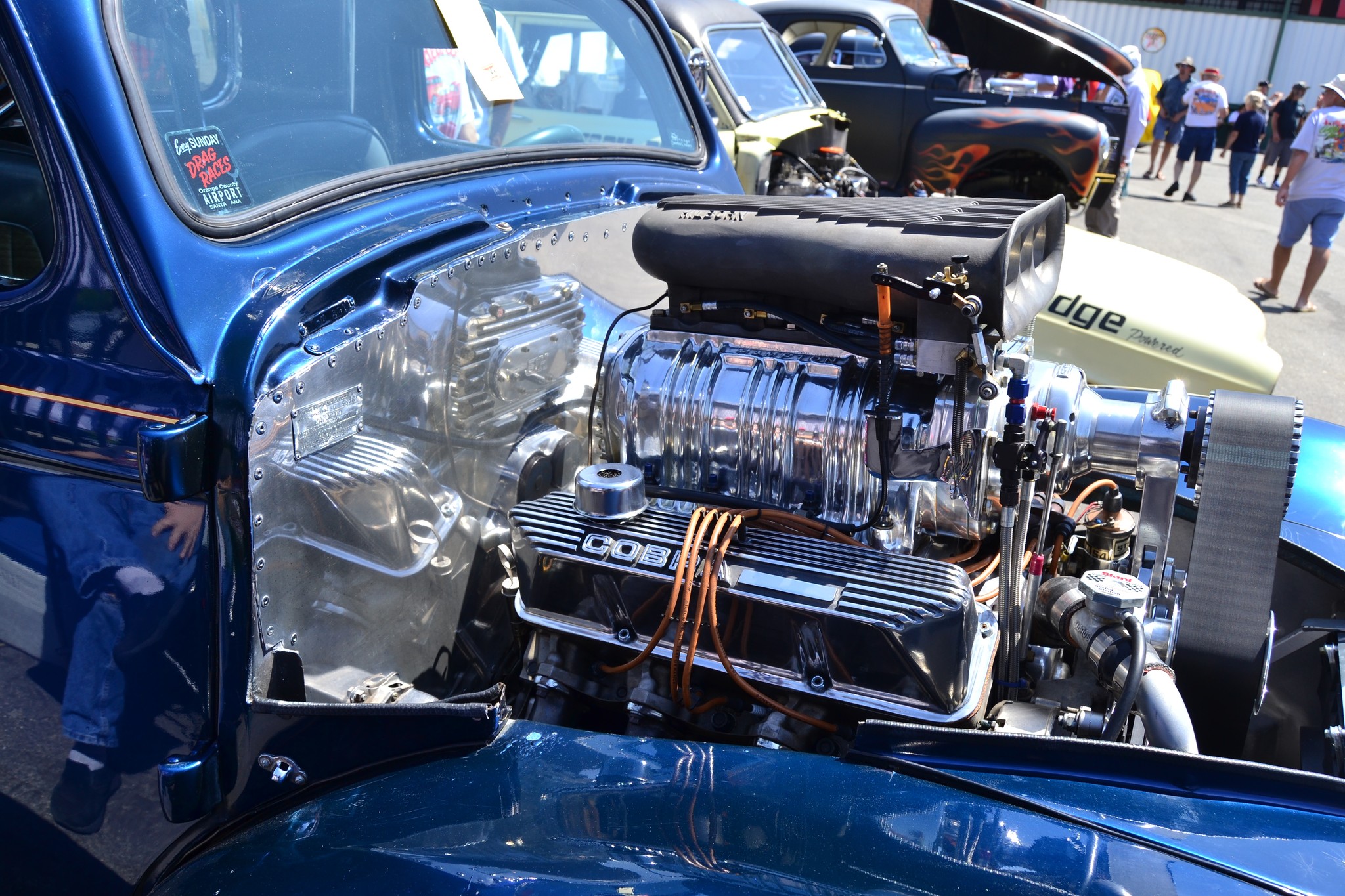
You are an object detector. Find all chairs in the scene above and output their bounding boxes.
[226,109,392,203]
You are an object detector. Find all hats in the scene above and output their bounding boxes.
[1293,83,1310,91]
[1320,74,1345,100]
[1258,81,1273,88]
[1175,56,1195,73]
[1200,67,1223,79]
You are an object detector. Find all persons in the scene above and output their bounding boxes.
[978,62,1106,103]
[1218,91,1266,207]
[1228,105,1245,123]
[1257,81,1283,147]
[1254,73,1345,314]
[30,472,208,834]
[423,1,530,147]
[1257,83,1311,189]
[1143,58,1198,179]
[1106,44,1151,221]
[1165,68,1230,202]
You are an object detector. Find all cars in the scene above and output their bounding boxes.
[0,0,1345,896]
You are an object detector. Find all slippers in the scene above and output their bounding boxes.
[1293,302,1318,312]
[1254,277,1280,299]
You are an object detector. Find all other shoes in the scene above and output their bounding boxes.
[1165,182,1179,196]
[1219,201,1242,208]
[1156,171,1166,180]
[1257,175,1266,184]
[1182,193,1196,201]
[1143,171,1154,179]
[1271,181,1281,190]
